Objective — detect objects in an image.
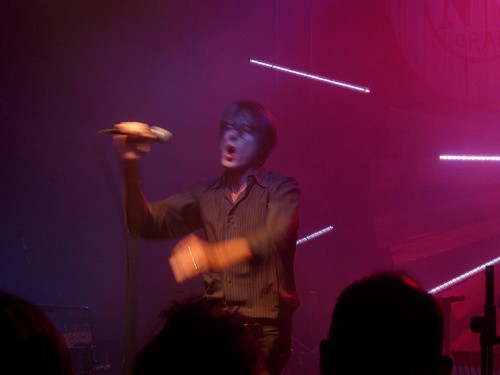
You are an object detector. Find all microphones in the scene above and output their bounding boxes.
[98,126,172,142]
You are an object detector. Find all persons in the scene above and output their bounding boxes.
[113,97,302,374]
[0,288,76,374]
[317,270,455,375]
[130,292,271,375]
